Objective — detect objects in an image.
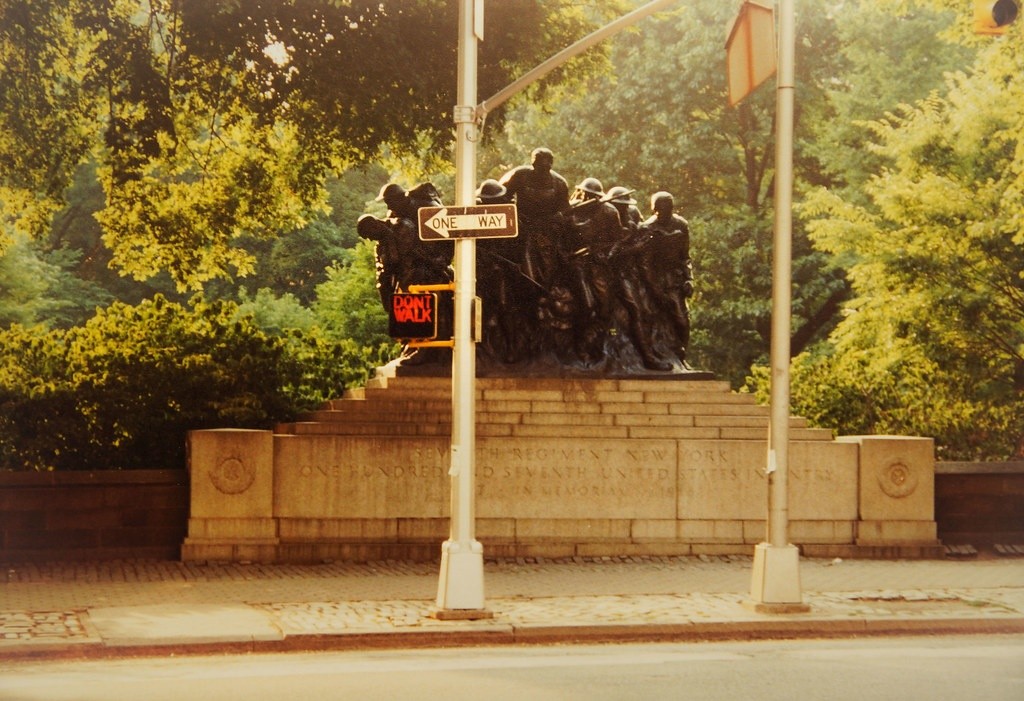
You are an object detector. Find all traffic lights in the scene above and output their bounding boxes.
[971,0,1020,36]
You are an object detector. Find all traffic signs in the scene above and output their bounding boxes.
[389,288,453,343]
[418,204,519,241]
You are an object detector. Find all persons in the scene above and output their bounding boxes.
[356,147,695,372]
